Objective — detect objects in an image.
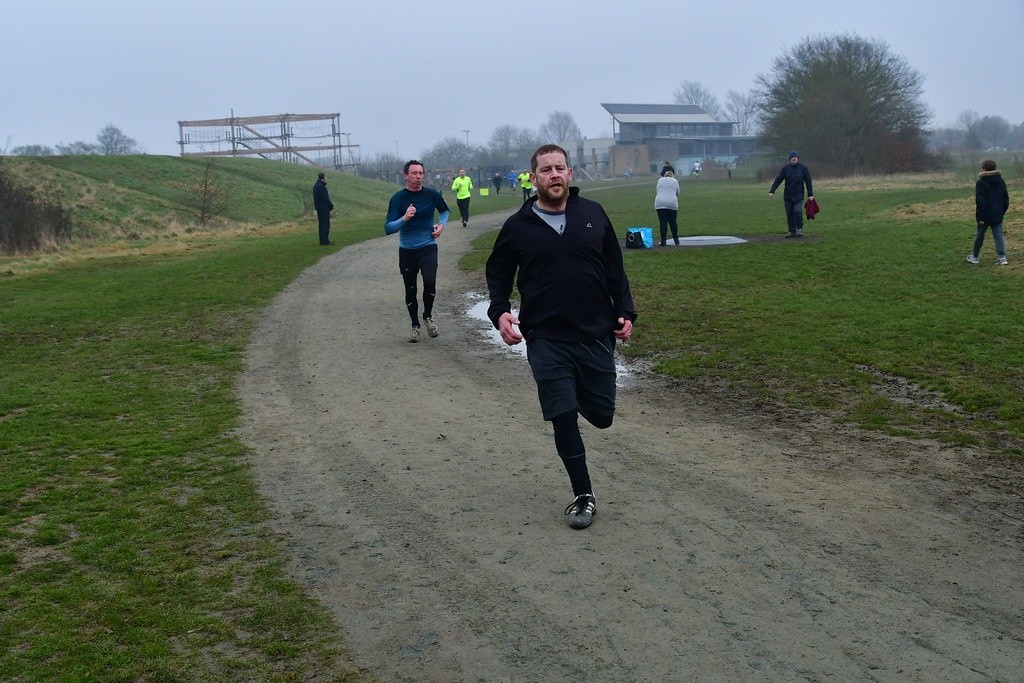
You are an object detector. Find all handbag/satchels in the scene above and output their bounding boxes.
[625,226,654,249]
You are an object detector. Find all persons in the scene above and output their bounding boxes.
[768,152,814,238]
[313,173,333,244]
[661,161,674,175]
[694,161,700,176]
[516,168,533,201]
[654,171,680,246]
[452,169,473,227]
[624,168,628,180]
[486,145,637,528]
[493,173,501,194]
[967,160,1009,264]
[384,160,450,342]
[508,170,517,193]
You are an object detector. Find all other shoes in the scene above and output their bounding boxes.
[322,240,333,245]
[408,327,422,343]
[424,316,440,338]
[795,228,803,235]
[786,233,794,237]
[463,221,466,227]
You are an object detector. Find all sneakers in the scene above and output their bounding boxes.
[966,254,979,263]
[995,256,1008,265]
[564,490,595,529]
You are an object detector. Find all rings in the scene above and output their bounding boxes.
[628,334,630,335]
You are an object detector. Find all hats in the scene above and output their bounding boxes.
[789,151,800,161]
